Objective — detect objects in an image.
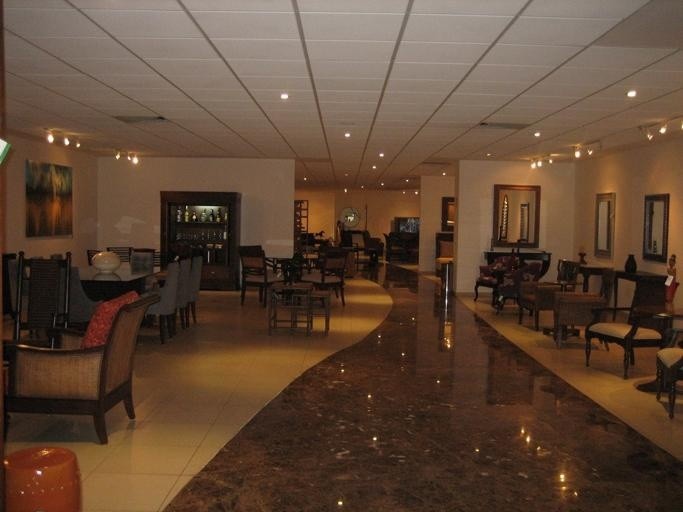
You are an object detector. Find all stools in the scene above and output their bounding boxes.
[4,447,82,512]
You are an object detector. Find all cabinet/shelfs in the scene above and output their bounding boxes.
[160,190,242,292]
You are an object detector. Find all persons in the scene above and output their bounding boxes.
[666,254,676,284]
[335,219,354,277]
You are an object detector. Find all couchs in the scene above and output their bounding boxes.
[435,233,453,276]
[383,232,419,263]
[340,230,384,256]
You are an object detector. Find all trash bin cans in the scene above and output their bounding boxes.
[435,257,456,293]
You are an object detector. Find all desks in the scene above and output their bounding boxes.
[484,252,552,278]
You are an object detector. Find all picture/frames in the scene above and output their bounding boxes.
[441,197,455,231]
[642,193,670,262]
[594,192,616,259]
[493,184,541,248]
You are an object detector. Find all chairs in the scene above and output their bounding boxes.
[1,251,72,366]
[2,246,203,347]
[2,290,162,445]
[300,233,315,246]
[238,238,355,336]
[474,257,683,420]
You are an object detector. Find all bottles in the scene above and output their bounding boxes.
[175,204,227,223]
[176,231,226,240]
